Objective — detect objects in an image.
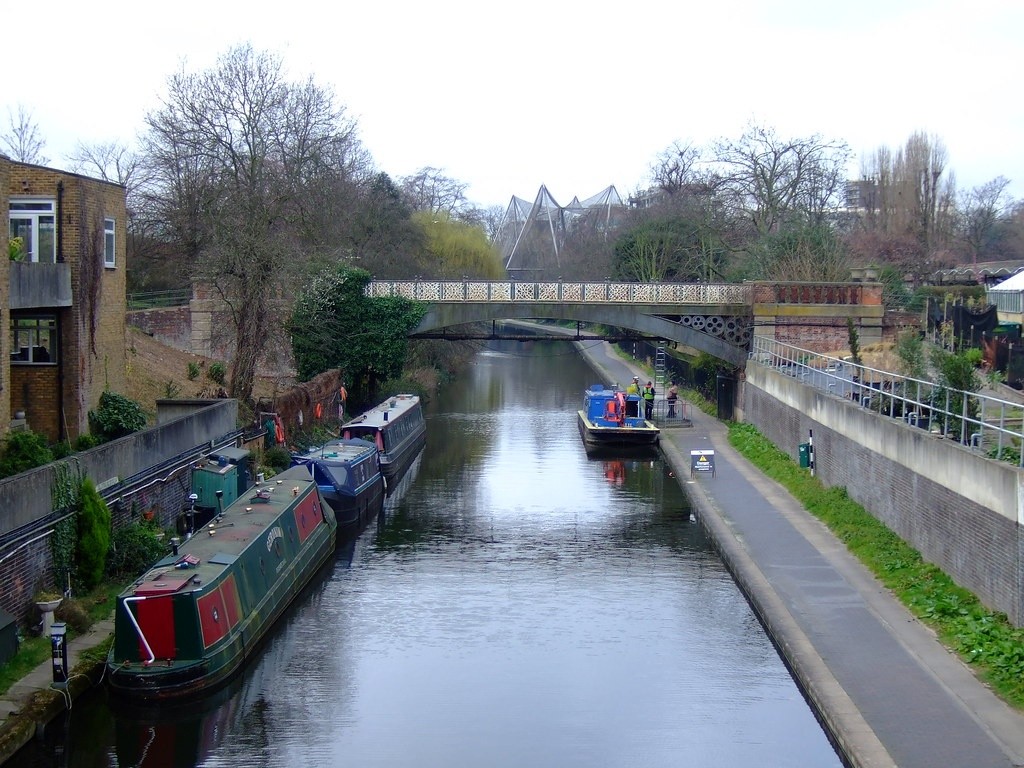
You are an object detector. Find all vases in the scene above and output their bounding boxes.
[35,598,64,611]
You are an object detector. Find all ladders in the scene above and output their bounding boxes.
[653,341,666,420]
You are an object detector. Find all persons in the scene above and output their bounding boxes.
[666,380,677,418]
[642,381,654,420]
[627,377,639,395]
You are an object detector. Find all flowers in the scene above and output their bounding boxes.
[33,591,62,602]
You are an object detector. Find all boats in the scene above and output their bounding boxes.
[340,392,426,478]
[577,384,660,455]
[107,462,338,709]
[288,439,387,534]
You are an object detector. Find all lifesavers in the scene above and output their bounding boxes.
[340,386,346,399]
[316,403,322,419]
[274,425,284,444]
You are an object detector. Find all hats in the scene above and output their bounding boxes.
[633,376,640,380]
